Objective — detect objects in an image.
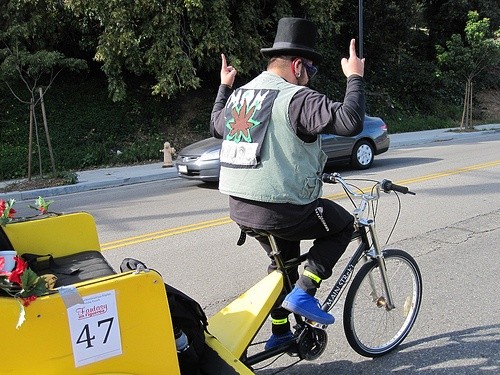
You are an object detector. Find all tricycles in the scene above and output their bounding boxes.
[0,169,425,375]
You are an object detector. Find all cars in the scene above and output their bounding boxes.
[175,110,389,190]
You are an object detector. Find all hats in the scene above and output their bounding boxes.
[260,17,323,59]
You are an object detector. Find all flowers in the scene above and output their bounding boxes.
[0,198,19,226]
[26,197,55,214]
[0,255,61,330]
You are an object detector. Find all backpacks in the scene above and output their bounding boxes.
[121,258,211,375]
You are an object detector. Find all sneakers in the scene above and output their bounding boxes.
[280,286,335,324]
[264,333,294,350]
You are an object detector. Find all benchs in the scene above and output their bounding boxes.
[0,211,181,375]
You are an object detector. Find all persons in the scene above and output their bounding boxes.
[210,17,365,352]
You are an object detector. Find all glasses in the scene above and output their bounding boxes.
[291,58,317,79]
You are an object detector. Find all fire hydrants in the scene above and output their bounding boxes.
[159,142,176,168]
